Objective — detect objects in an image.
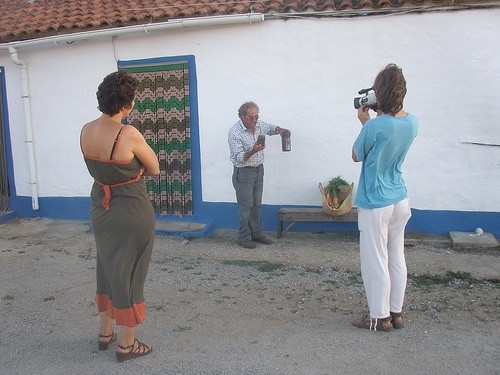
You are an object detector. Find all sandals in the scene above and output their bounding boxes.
[391,311,405,329]
[352,314,394,332]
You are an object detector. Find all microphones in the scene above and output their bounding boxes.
[358,87,373,94]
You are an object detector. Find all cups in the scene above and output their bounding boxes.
[282,131,292,151]
[257,135,266,148]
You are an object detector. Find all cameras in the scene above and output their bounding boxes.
[354,91,378,112]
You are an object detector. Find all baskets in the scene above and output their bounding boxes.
[318,180,354,217]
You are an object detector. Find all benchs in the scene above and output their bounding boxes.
[278,208,358,240]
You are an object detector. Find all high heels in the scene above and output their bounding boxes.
[97,331,117,351]
[115,337,152,363]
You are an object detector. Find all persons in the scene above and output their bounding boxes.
[351,63,420,331]
[79,71,159,362]
[228,102,291,249]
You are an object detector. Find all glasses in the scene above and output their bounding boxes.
[245,114,260,120]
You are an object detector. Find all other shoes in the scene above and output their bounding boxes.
[254,234,273,245]
[238,239,257,249]
